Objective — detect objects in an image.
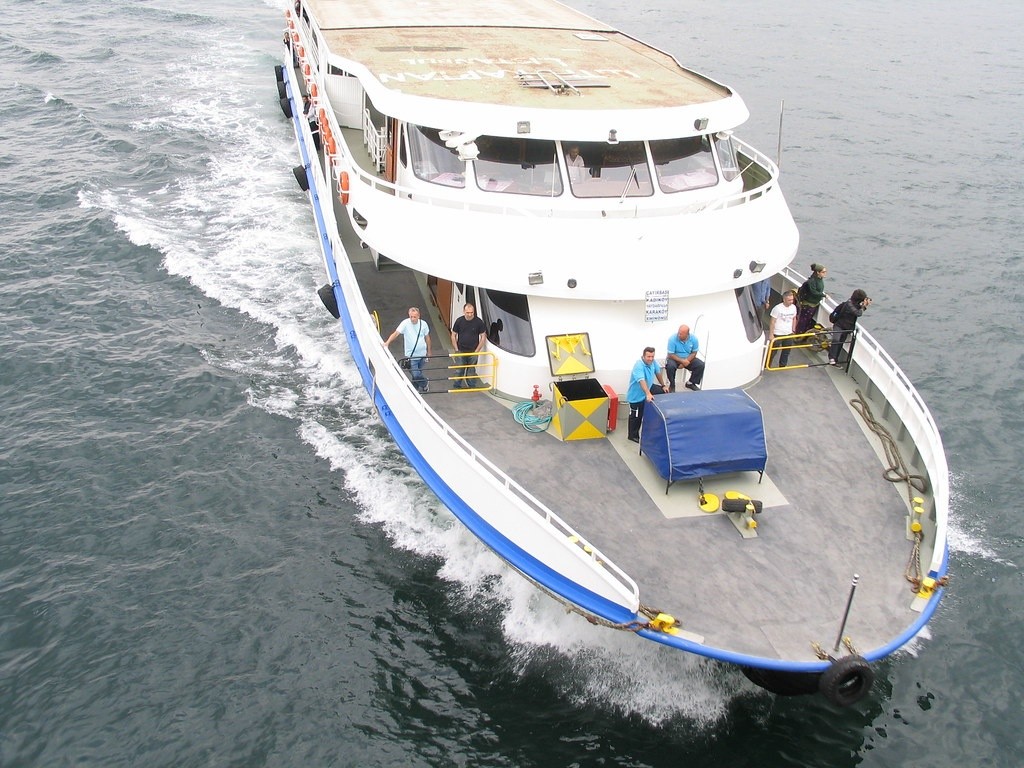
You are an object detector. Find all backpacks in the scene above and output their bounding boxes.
[829,300,851,323]
[798,279,816,302]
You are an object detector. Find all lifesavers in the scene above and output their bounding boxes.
[279,98,293,119]
[287,10,290,26]
[317,283,340,319]
[274,65,283,81]
[294,34,299,48]
[311,85,317,106]
[289,21,294,38]
[304,64,310,83]
[318,109,337,167]
[339,172,350,205]
[293,165,309,191]
[277,81,288,99]
[300,47,305,64]
[821,656,875,706]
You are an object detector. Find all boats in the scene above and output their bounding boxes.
[274,2,951,708]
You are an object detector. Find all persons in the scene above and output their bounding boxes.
[752,278,771,330]
[627,346,668,443]
[666,324,704,392]
[306,94,320,150]
[385,307,431,395]
[795,264,826,342]
[303,95,310,114]
[451,303,487,388]
[566,144,585,185]
[765,291,797,367]
[829,289,872,369]
[283,32,290,50]
[295,0,309,23]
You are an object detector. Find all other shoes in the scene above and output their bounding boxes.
[669,385,675,393]
[829,358,835,364]
[685,382,700,391]
[628,437,639,443]
[421,378,429,392]
[832,365,843,369]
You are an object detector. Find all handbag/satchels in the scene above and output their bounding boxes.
[402,359,411,369]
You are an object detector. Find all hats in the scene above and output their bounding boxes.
[811,263,824,272]
